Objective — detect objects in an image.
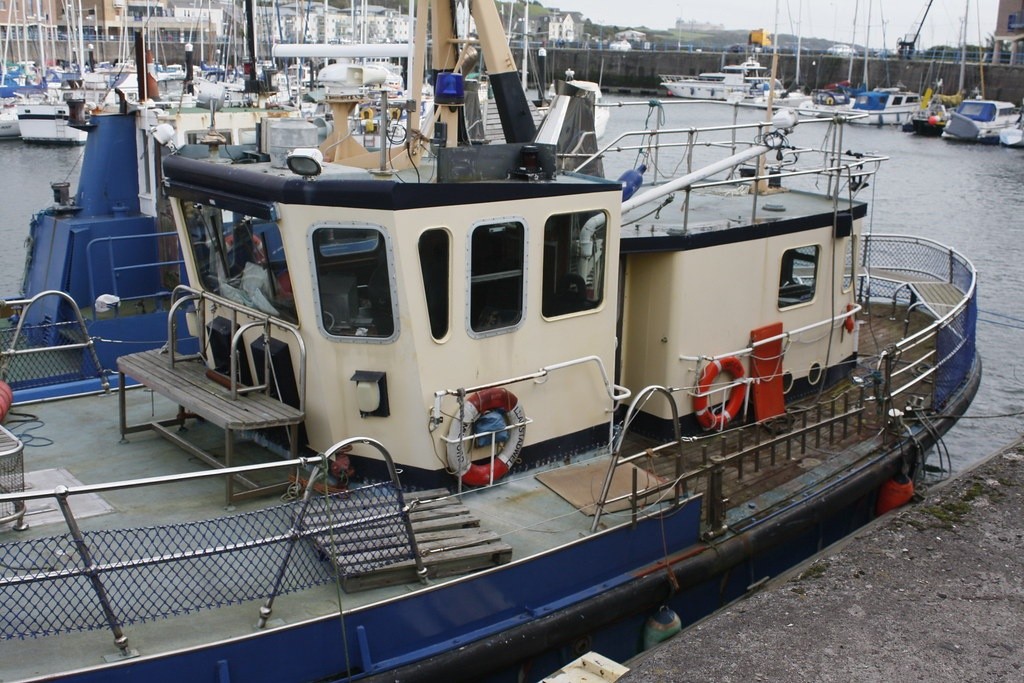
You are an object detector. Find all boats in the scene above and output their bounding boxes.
[1,0,984,683]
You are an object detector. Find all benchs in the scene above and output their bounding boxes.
[114,285,305,508]
[790,263,967,340]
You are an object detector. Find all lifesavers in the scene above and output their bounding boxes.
[389,108,401,120]
[695,358,748,430]
[447,388,527,486]
[223,233,265,268]
[361,107,374,119]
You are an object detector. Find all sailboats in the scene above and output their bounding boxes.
[0,1,610,148]
[657,0,1024,151]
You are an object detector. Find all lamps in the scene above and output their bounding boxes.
[149,123,177,156]
[286,148,324,181]
